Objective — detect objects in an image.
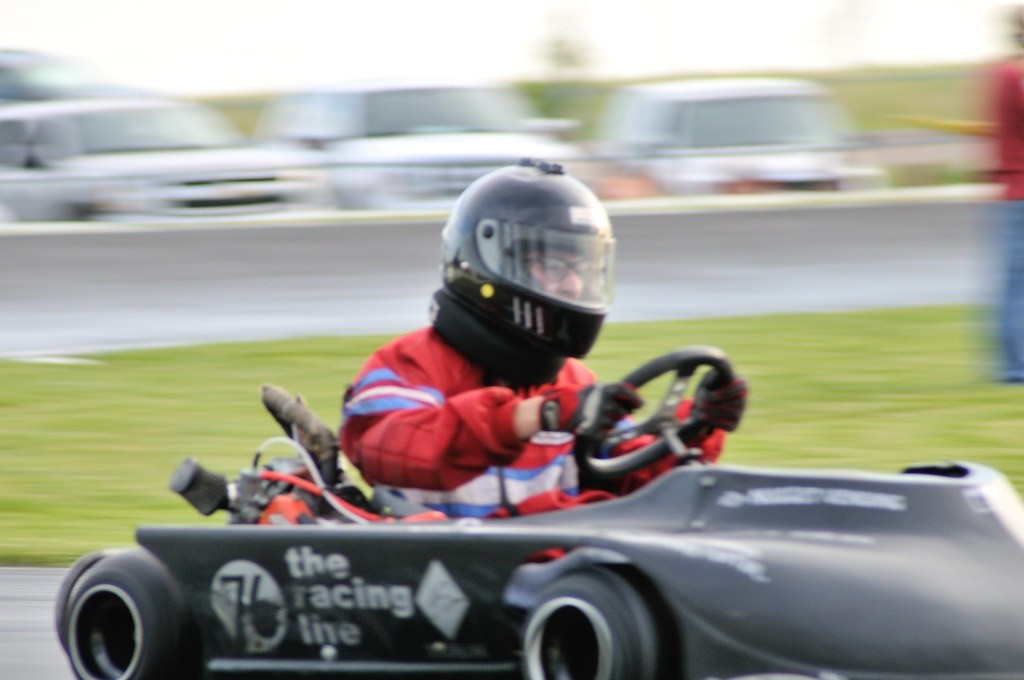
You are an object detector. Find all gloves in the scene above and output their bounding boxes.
[692,369,747,431]
[540,380,644,441]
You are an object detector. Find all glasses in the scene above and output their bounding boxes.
[509,250,606,292]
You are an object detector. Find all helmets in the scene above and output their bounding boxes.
[440,165,616,362]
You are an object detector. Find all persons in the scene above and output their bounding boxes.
[336,157,746,564]
[987,0,1024,382]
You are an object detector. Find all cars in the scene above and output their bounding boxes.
[583,75,895,202]
[0,96,336,222]
[267,79,594,213]
